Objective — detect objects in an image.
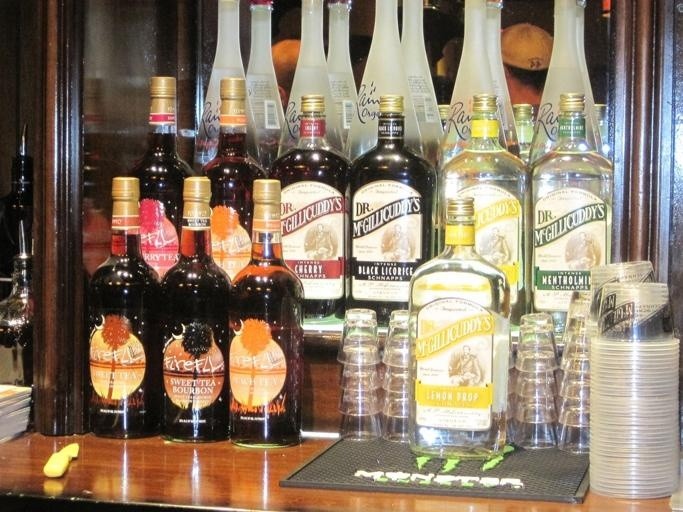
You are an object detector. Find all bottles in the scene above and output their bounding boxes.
[344,0,426,164]
[246,2,286,171]
[401,0,446,168]
[529,92,612,333]
[194,0,248,166]
[0,254,34,435]
[325,3,358,145]
[435,0,522,174]
[438,93,534,328]
[276,0,346,160]
[268,95,353,320]
[344,94,437,327]
[407,195,511,462]
[124,77,197,254]
[154,176,231,443]
[85,176,163,440]
[228,180,306,449]
[0,155,36,302]
[527,1,607,168]
[197,77,268,243]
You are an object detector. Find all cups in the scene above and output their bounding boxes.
[514,313,556,448]
[338,308,381,440]
[378,310,411,445]
[556,293,593,452]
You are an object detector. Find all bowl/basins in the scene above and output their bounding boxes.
[588,263,679,500]
